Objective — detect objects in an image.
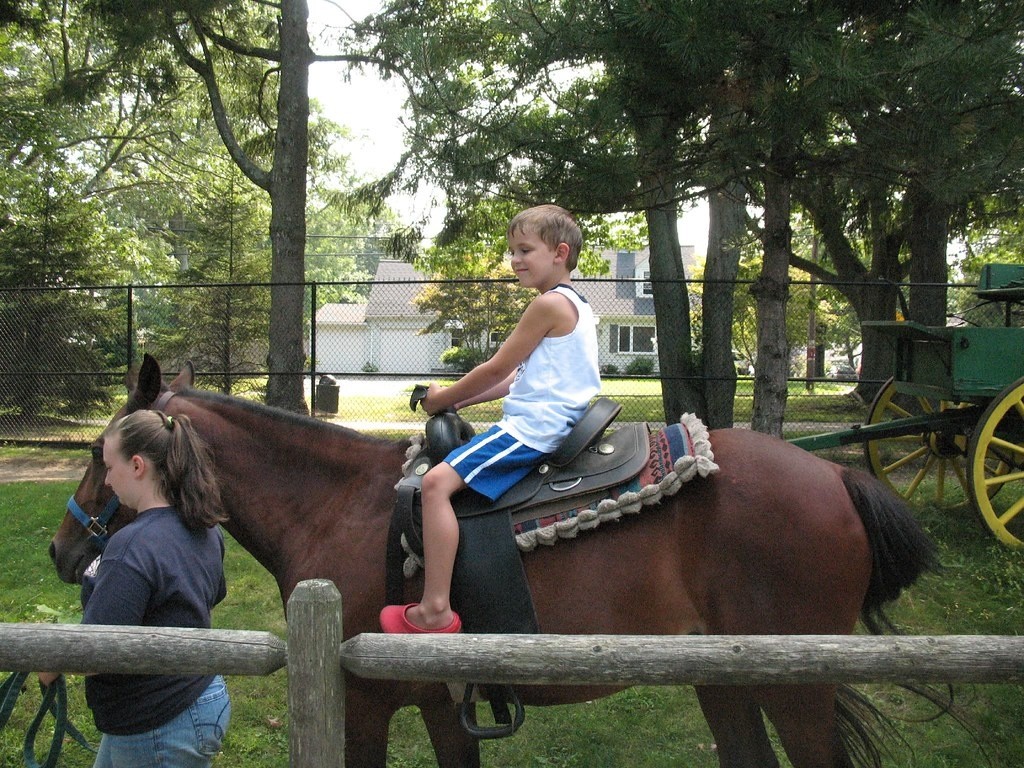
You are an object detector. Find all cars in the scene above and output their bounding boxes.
[830,359,859,381]
[733,351,750,375]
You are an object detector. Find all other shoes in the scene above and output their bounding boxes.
[379,603,461,633]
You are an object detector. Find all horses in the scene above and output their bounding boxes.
[48,352,1024,768]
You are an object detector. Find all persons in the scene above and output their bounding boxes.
[746,361,755,376]
[37,411,230,768]
[380,205,601,633]
